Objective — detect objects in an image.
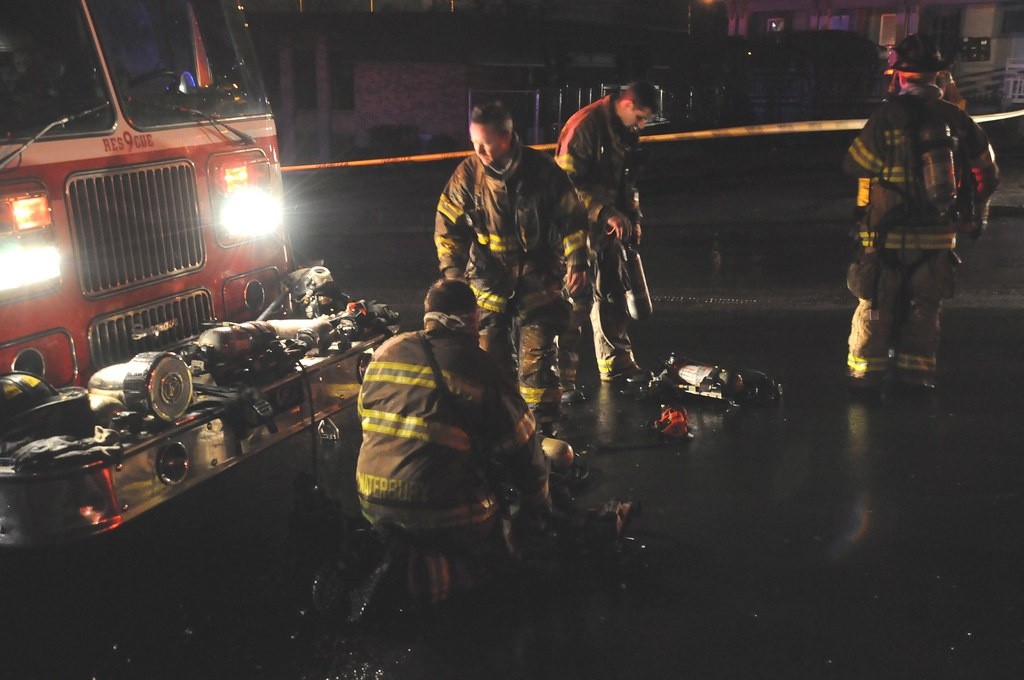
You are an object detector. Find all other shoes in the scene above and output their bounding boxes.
[533,405,559,422]
[841,377,878,399]
[625,370,649,383]
[560,388,586,405]
[900,373,937,394]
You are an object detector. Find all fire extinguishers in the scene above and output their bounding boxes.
[915,114,960,221]
[613,233,653,321]
[647,351,730,398]
[538,432,575,469]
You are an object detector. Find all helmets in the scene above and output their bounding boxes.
[883,33,942,76]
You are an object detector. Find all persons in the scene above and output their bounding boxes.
[827,32,1000,403]
[356,280,536,611]
[557,82,664,403]
[434,100,589,443]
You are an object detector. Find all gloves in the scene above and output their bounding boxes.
[967,219,987,241]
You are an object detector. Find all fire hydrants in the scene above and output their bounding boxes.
[1,1,400,547]
